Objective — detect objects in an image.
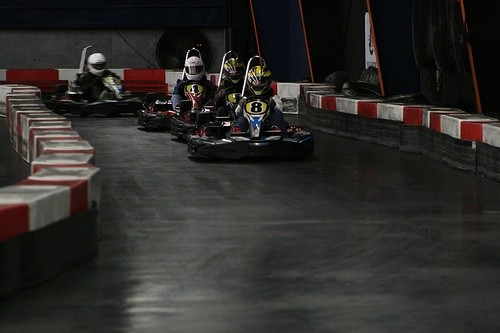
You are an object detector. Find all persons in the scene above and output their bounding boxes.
[210,57,246,116]
[72,52,123,101]
[229,65,291,135]
[172,56,216,123]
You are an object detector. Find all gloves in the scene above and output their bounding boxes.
[239,98,246,108]
[267,98,276,107]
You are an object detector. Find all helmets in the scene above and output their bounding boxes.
[182,57,205,81]
[246,66,273,96]
[87,52,107,76]
[222,58,246,85]
